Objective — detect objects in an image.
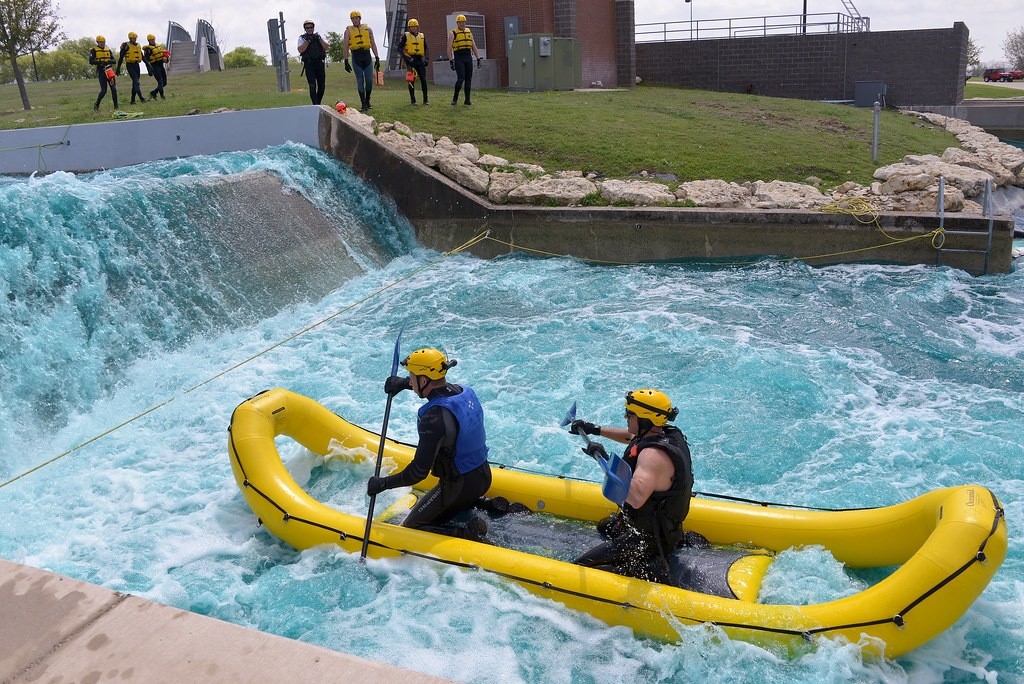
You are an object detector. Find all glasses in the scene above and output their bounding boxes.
[305,26,314,29]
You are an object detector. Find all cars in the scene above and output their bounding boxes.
[1009,70,1024,79]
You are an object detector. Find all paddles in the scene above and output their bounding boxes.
[560,401,631,507]
[360,321,407,564]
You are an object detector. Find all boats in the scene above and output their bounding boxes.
[228,386,1009,663]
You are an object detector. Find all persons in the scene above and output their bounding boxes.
[367,348,530,546]
[343,10,380,112]
[397,18,430,106]
[297,20,330,105]
[89,35,118,112]
[570,389,709,586]
[115,32,153,104]
[142,34,169,99]
[447,15,481,106]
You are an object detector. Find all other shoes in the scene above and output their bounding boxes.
[424,100,429,105]
[464,101,473,106]
[94,91,166,112]
[411,101,419,107]
[359,102,373,113]
[451,98,457,104]
[681,529,710,549]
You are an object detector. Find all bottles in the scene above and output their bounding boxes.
[185,108,199,115]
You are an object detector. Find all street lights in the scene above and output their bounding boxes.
[685,0,693,41]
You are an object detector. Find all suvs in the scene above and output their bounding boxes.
[983,69,1013,83]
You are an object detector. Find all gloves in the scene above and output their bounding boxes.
[368,474,386,498]
[476,58,481,70]
[373,57,380,69]
[450,60,455,71]
[385,375,409,395]
[410,60,415,66]
[149,69,155,75]
[115,68,120,77]
[344,59,353,74]
[424,60,428,66]
[570,418,611,463]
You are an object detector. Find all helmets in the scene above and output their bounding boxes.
[406,349,448,382]
[408,18,420,26]
[456,14,466,23]
[350,11,362,19]
[625,386,671,425]
[128,32,138,38]
[96,35,105,42]
[147,34,155,40]
[303,20,314,27]
